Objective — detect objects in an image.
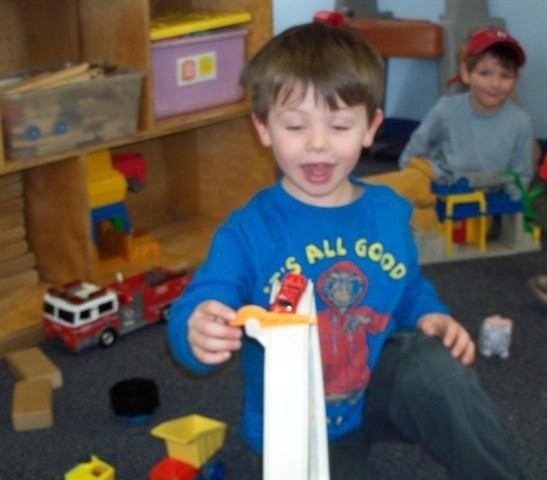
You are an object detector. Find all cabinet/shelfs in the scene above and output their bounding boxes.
[0,1,261,362]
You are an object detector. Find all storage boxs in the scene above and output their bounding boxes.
[0,59,148,160]
[148,8,252,121]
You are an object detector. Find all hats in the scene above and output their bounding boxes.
[462,25,526,68]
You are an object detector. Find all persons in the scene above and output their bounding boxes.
[166,17,539,480]
[395,22,536,237]
